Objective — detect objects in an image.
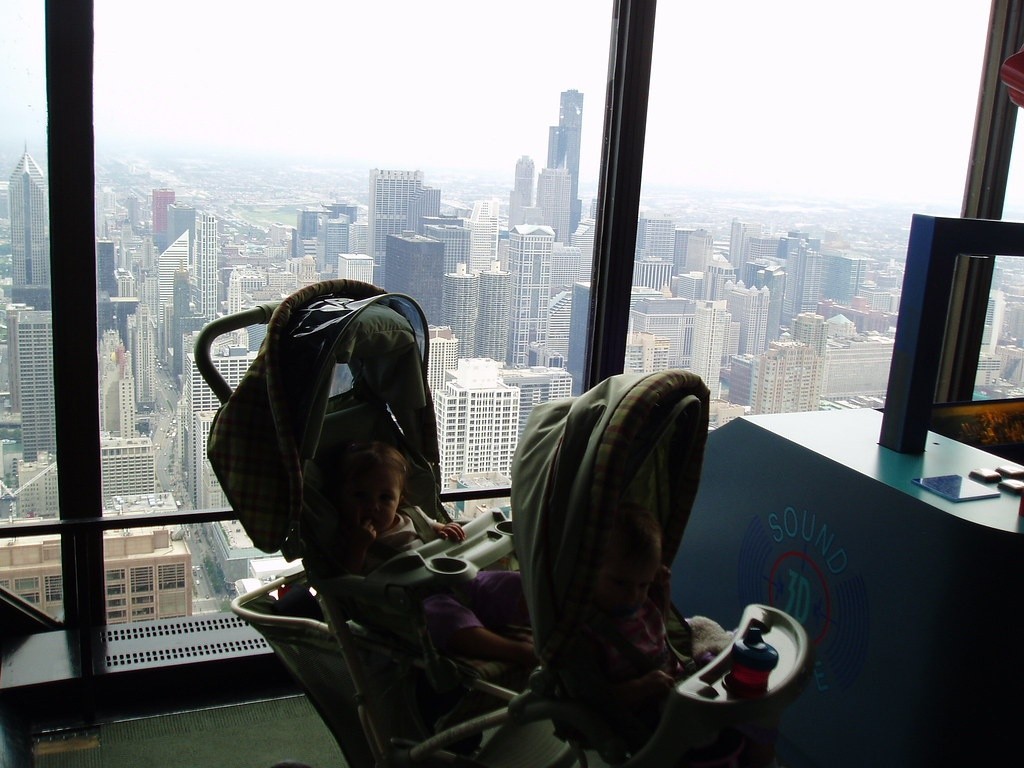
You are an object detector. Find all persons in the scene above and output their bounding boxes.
[586,502,777,768]
[328,438,537,669]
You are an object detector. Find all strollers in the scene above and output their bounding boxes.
[194,278,800,768]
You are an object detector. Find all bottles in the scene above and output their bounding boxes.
[724,627,777,699]
